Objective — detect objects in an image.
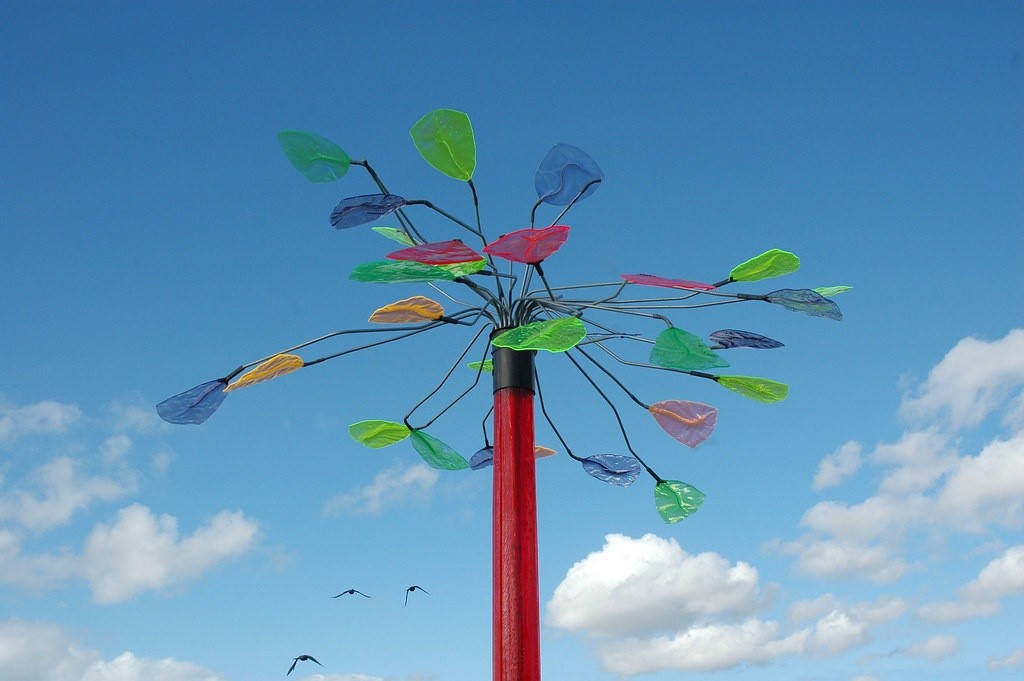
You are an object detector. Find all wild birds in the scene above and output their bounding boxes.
[286,654,326,676]
[404,585,432,607]
[330,588,372,601]
